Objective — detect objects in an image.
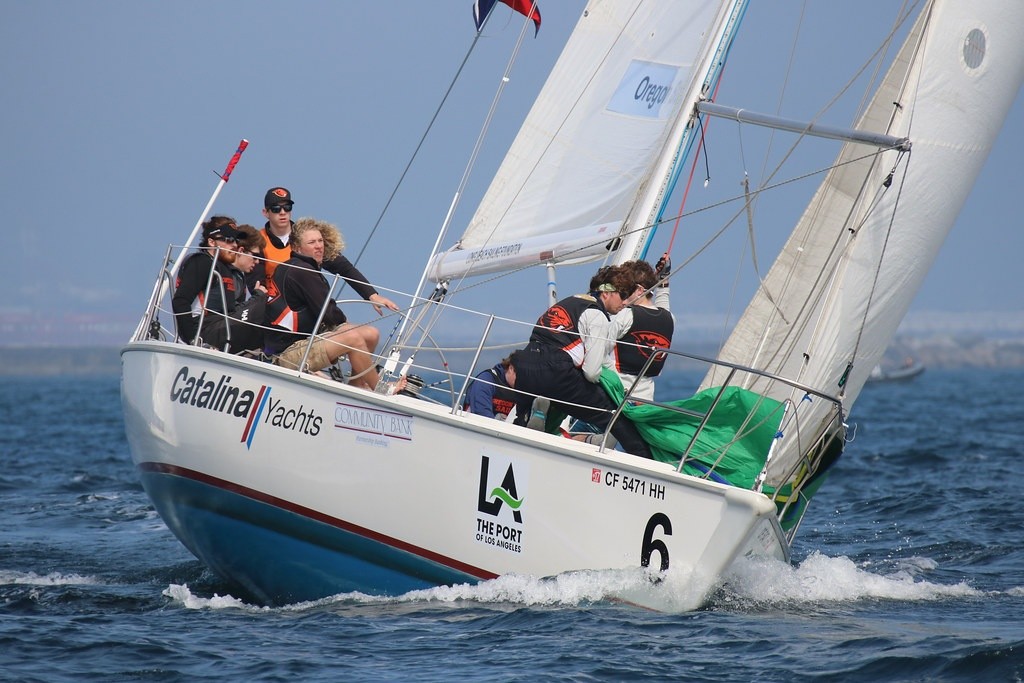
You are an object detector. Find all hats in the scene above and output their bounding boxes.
[265,187,294,208]
[203,222,248,240]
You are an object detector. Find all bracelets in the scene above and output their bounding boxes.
[659,283,669,287]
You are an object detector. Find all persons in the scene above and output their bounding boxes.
[173,187,400,379]
[463,252,675,450]
[265,221,407,394]
[515,266,655,460]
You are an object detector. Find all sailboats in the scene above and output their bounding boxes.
[121,0,1024,613]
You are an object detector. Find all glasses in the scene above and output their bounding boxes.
[212,235,240,244]
[244,248,260,261]
[619,291,628,300]
[268,204,292,213]
